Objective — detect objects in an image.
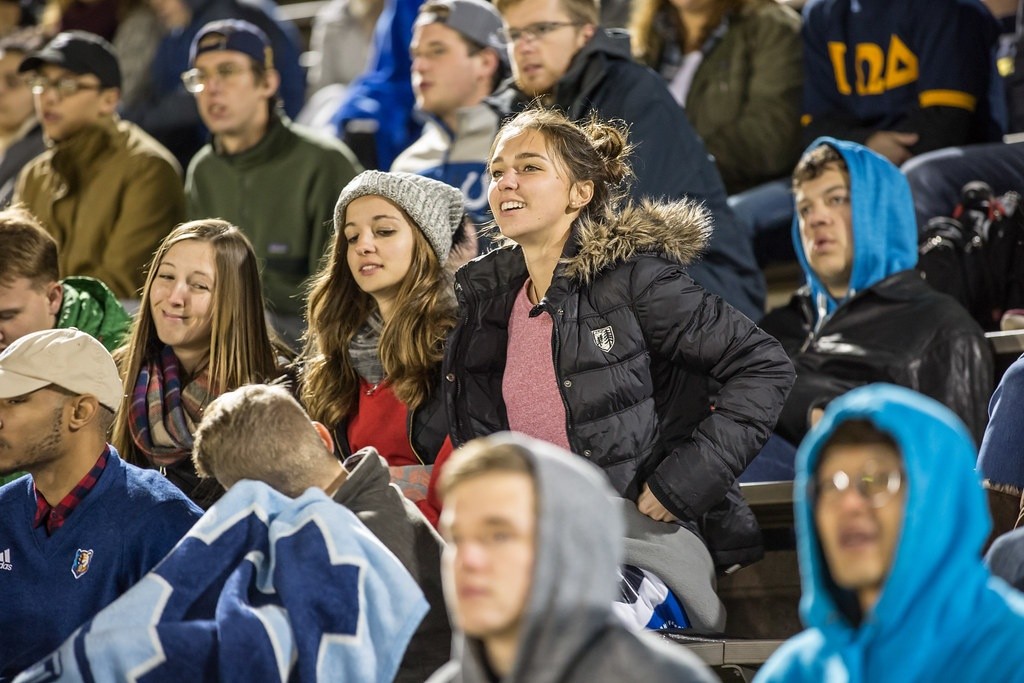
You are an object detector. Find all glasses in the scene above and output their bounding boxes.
[503,17,591,45]
[813,465,911,503]
[182,65,258,93]
[24,74,110,96]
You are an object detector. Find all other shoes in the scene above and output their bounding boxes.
[915,217,964,275]
[962,180,1011,255]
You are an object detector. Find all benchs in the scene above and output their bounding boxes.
[667,479,797,671]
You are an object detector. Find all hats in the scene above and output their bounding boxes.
[17,29,121,89]
[0,327,125,412]
[188,18,273,77]
[412,0,510,67]
[333,168,464,269]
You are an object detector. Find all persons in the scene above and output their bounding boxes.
[443,109,797,632]
[190,382,452,683]
[0,0,1024,592]
[751,382,1024,683]
[422,431,720,683]
[0,326,205,683]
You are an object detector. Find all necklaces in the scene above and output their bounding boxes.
[534,285,540,304]
